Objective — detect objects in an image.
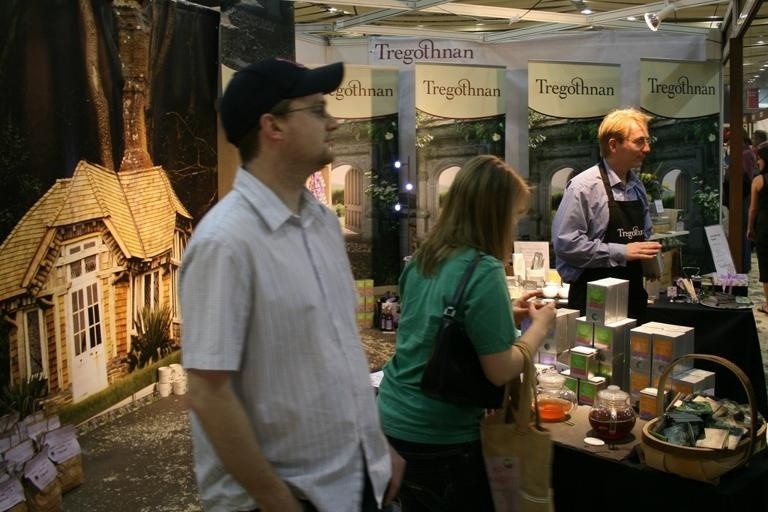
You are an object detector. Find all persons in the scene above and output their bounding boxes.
[377,154,559,511]
[176,56,407,512]
[550,108,663,327]
[722,125,767,314]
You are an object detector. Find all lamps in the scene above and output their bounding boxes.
[643,3,677,35]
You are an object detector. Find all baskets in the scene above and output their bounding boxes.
[640,353,767,483]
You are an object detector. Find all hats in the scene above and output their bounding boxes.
[219,59,344,148]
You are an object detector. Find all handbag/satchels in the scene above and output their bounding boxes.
[479,340,556,512]
[420,253,505,409]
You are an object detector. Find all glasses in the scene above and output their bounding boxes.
[271,101,327,120]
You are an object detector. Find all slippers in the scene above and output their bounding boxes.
[757,305,768,315]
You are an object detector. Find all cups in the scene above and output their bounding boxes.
[157,363,188,397]
[682,265,701,280]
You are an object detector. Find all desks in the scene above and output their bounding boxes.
[549,435,768,512]
[644,290,754,403]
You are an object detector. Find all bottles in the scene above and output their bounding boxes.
[690,273,702,294]
[530,368,579,421]
[589,383,637,442]
[379,311,393,330]
